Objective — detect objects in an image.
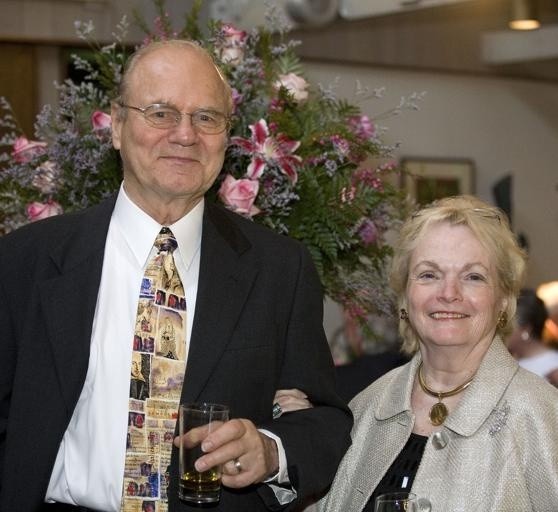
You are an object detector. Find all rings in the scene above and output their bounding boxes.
[271,403,281,417]
[232,457,244,474]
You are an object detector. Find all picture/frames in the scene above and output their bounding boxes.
[398,153,478,218]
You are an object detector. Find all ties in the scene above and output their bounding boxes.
[118,228,187,512]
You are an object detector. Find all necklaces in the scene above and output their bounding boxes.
[418,360,478,425]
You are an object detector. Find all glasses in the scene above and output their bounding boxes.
[121,104,232,135]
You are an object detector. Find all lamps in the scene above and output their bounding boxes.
[504,1,546,34]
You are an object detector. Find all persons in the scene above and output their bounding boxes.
[2,36,356,511]
[271,193,556,511]
[512,229,547,344]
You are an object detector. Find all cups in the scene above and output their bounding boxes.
[373,491,421,510]
[178,401,231,503]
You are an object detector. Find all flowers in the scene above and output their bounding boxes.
[0,1,431,370]
